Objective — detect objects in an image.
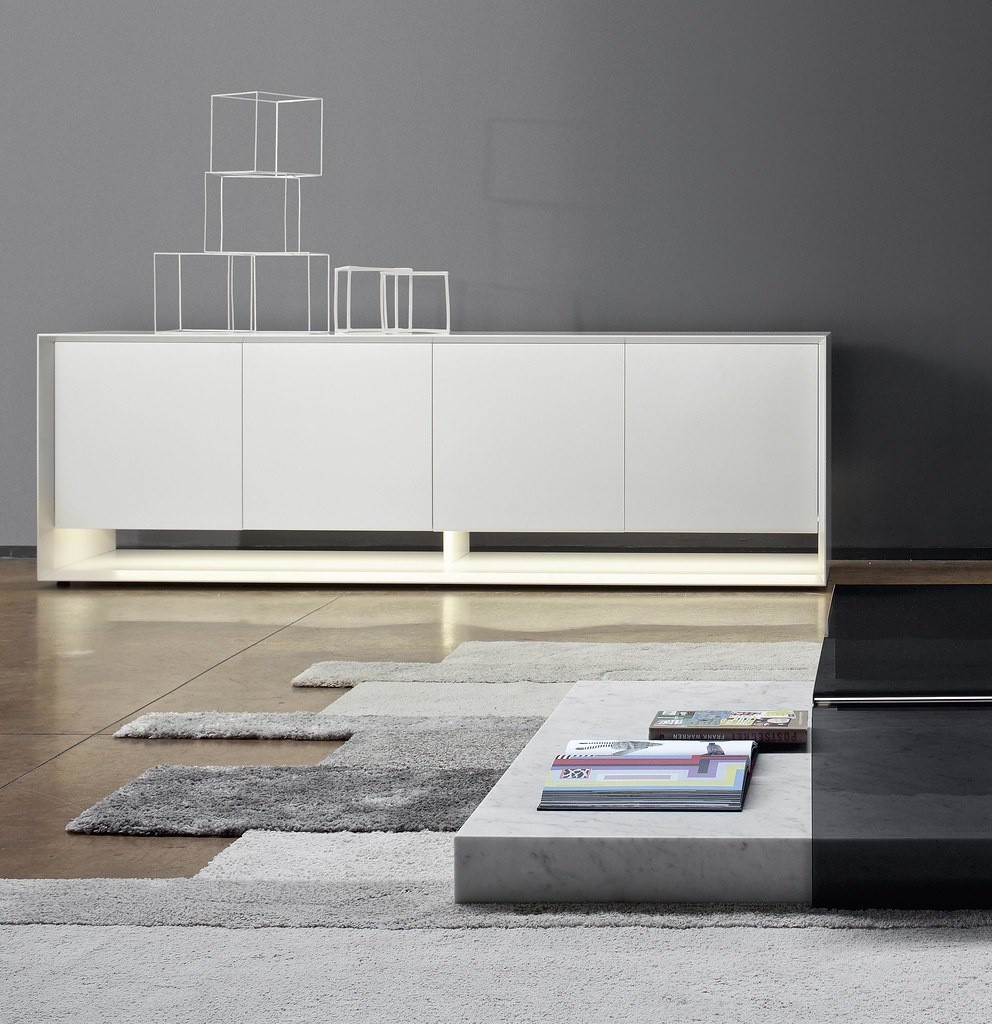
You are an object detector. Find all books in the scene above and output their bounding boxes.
[648,710,808,744]
[537,740,758,812]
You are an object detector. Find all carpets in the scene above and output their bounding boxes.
[190,828,455,882]
[0,924,992,1024]
[292,641,821,687]
[0,876,991,930]
[113,711,543,770]
[64,762,507,832]
[318,682,572,718]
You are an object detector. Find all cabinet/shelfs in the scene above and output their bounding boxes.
[37,329,830,592]
